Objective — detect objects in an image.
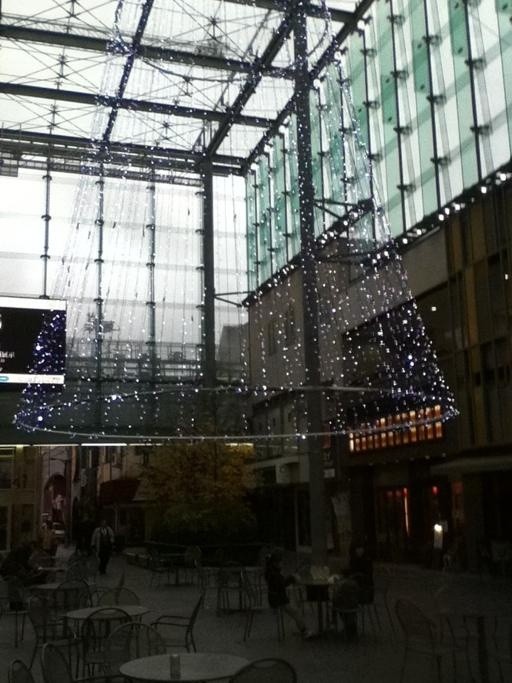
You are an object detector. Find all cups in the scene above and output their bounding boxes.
[169,653,181,681]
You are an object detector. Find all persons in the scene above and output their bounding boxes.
[39,519,57,551]
[91,519,115,574]
[338,538,373,640]
[264,550,316,640]
[1,539,38,611]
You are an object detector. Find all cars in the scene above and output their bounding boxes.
[53,522,67,543]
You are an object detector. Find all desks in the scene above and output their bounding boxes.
[61,602,162,657]
[114,651,250,680]
[438,608,507,679]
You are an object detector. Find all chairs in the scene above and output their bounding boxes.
[393,599,465,680]
[8,660,34,680]
[10,542,126,648]
[231,657,299,681]
[241,561,395,646]
[489,649,512,682]
[143,543,229,588]
[40,639,74,682]
[20,591,80,670]
[97,587,142,659]
[103,620,168,680]
[78,606,132,678]
[147,589,207,657]
